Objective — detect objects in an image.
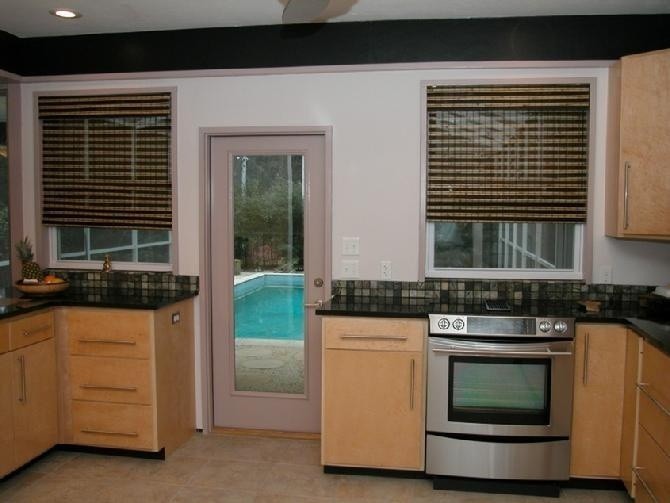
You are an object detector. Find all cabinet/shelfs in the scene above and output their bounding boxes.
[321,316,425,477]
[60,296,198,462]
[570,324,628,479]
[1,309,59,482]
[604,46,669,243]
[621,332,670,503]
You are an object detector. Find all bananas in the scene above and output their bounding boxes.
[51,278,65,284]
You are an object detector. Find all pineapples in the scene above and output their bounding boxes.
[12,236,44,282]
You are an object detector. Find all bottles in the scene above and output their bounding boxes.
[102,253,112,272]
[12,277,71,295]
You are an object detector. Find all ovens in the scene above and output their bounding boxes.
[425,314,575,481]
